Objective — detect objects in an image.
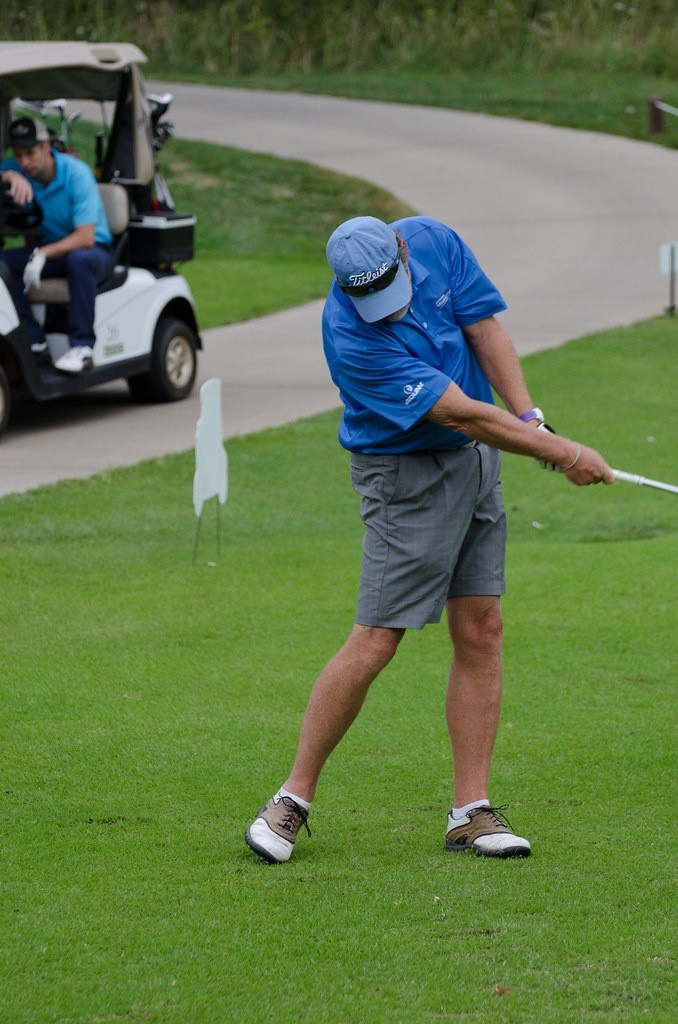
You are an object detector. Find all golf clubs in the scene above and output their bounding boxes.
[610,470,678,496]
[49,99,83,150]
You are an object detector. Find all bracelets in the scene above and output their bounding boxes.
[563,443,580,470]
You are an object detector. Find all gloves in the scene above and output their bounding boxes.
[23,248,46,293]
[534,422,563,473]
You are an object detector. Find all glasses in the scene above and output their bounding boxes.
[334,248,401,296]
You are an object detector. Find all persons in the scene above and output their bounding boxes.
[0,115,113,372]
[243,215,615,864]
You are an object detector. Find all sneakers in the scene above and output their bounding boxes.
[244,796,312,864]
[55,343,96,375]
[444,803,533,859]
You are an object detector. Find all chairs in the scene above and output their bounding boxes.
[29,181,132,305]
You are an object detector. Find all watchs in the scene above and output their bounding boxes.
[519,408,544,423]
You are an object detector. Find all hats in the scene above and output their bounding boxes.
[328,214,414,322]
[5,115,50,146]
